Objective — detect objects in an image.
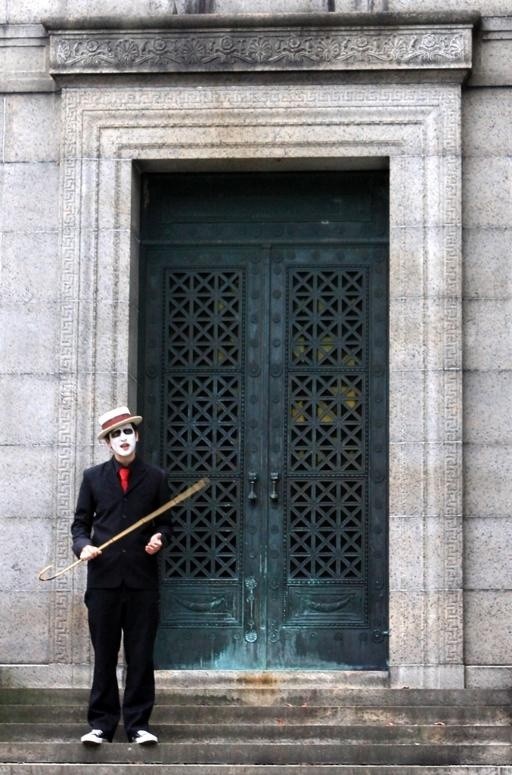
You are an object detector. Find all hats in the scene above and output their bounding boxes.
[96,407,143,439]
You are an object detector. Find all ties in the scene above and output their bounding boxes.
[119,467,129,493]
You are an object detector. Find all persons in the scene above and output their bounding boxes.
[69,405,173,748]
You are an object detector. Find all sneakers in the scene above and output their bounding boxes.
[131,730,158,744]
[81,729,108,744]
